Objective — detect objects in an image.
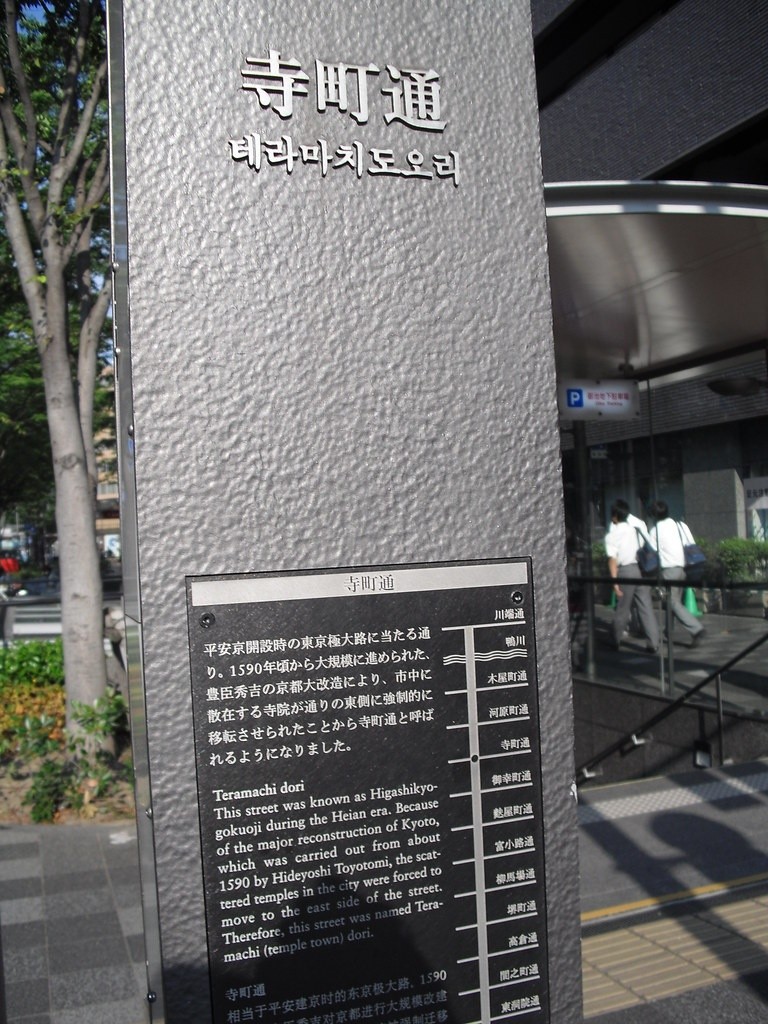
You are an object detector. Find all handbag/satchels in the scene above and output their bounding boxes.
[675,519,707,569]
[634,526,661,576]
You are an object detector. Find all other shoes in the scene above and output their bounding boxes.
[604,639,618,651]
[690,629,707,647]
[646,645,658,654]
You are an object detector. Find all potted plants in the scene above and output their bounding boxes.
[693,537,768,618]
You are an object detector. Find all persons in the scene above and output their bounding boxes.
[604,498,664,653]
[648,500,708,648]
